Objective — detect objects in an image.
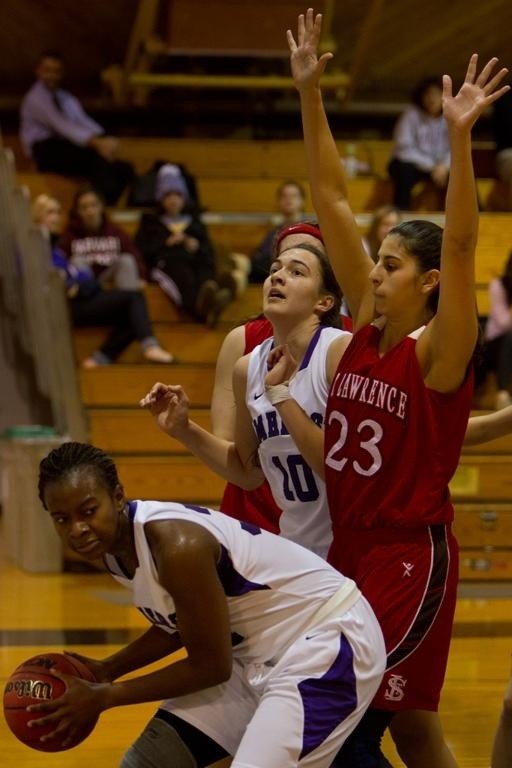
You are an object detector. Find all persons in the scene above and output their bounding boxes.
[385,76,452,213]
[484,241,512,403]
[287,7,511,768]
[211,223,356,532]
[463,405,512,768]
[135,162,225,331]
[33,194,175,369]
[26,444,388,768]
[139,243,354,561]
[57,191,146,290]
[19,53,147,209]
[227,180,317,295]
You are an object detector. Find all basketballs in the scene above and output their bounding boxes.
[4,654,101,753]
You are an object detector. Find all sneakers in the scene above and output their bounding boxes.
[140,343,174,365]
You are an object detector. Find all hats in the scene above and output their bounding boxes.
[152,162,189,202]
